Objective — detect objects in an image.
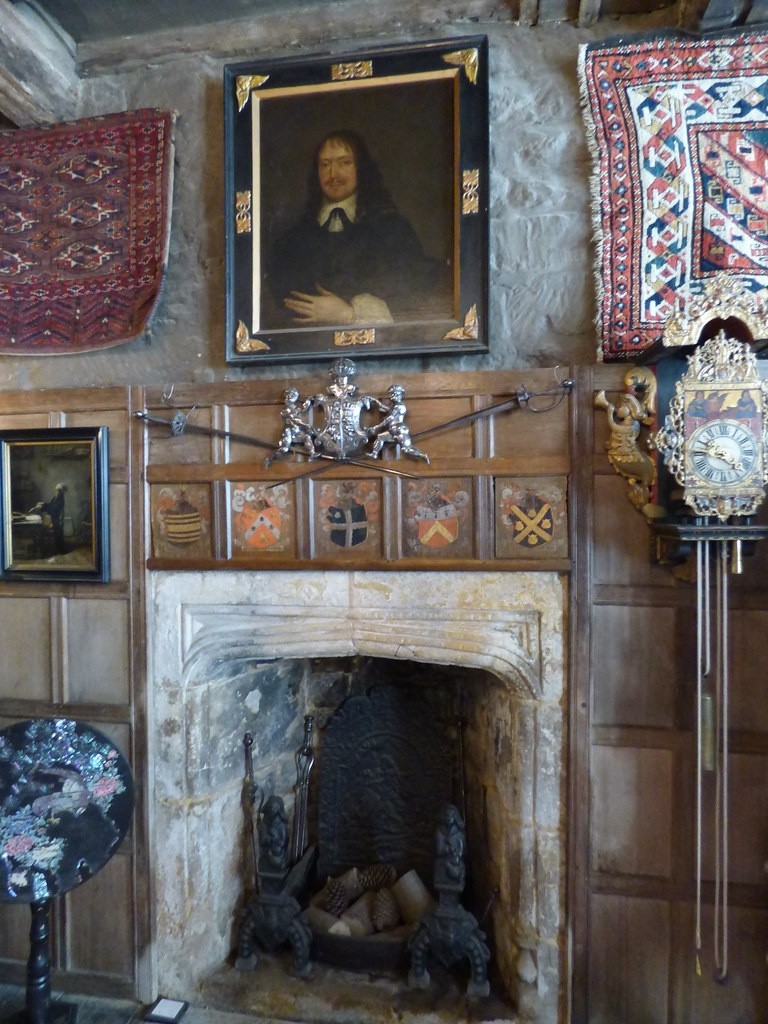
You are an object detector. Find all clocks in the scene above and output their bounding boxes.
[647,330,768,521]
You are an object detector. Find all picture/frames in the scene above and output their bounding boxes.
[0,428,110,584]
[216,33,490,362]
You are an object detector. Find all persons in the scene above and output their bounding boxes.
[606,395,644,454]
[261,793,291,866]
[688,390,705,416]
[272,128,425,324]
[435,805,467,891]
[263,386,323,468]
[31,483,68,553]
[312,358,392,458]
[738,390,758,420]
[371,384,434,464]
